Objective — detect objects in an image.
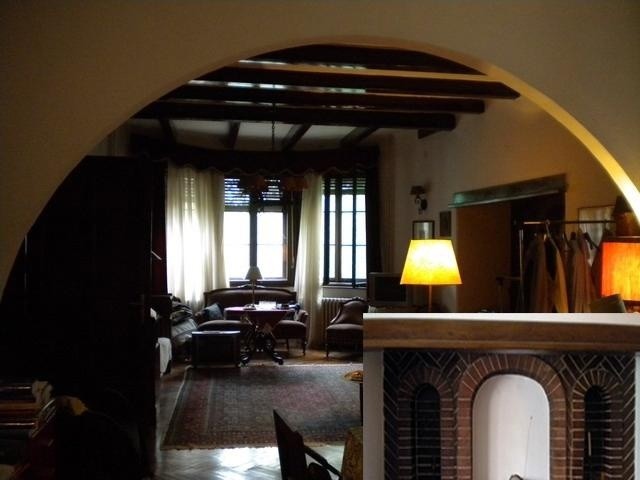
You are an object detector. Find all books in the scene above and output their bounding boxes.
[0,383,59,439]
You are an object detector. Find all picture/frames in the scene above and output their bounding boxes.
[412,220,434,240]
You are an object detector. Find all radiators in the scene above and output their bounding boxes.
[320,297,360,346]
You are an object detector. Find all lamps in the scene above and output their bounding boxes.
[237,101,314,195]
[599,236,640,305]
[409,184,428,215]
[400,240,463,313]
[246,266,262,308]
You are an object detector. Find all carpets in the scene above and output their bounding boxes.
[159,359,365,452]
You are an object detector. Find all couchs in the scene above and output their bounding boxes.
[324,298,370,360]
[192,287,307,355]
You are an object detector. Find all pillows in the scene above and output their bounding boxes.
[203,302,223,322]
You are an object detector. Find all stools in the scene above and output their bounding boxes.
[190,330,241,369]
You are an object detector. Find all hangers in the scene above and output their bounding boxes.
[518,219,613,312]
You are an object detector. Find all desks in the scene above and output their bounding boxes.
[223,305,296,365]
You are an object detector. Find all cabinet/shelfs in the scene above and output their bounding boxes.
[0,155,158,479]
[0,380,58,480]
[370,307,417,314]
